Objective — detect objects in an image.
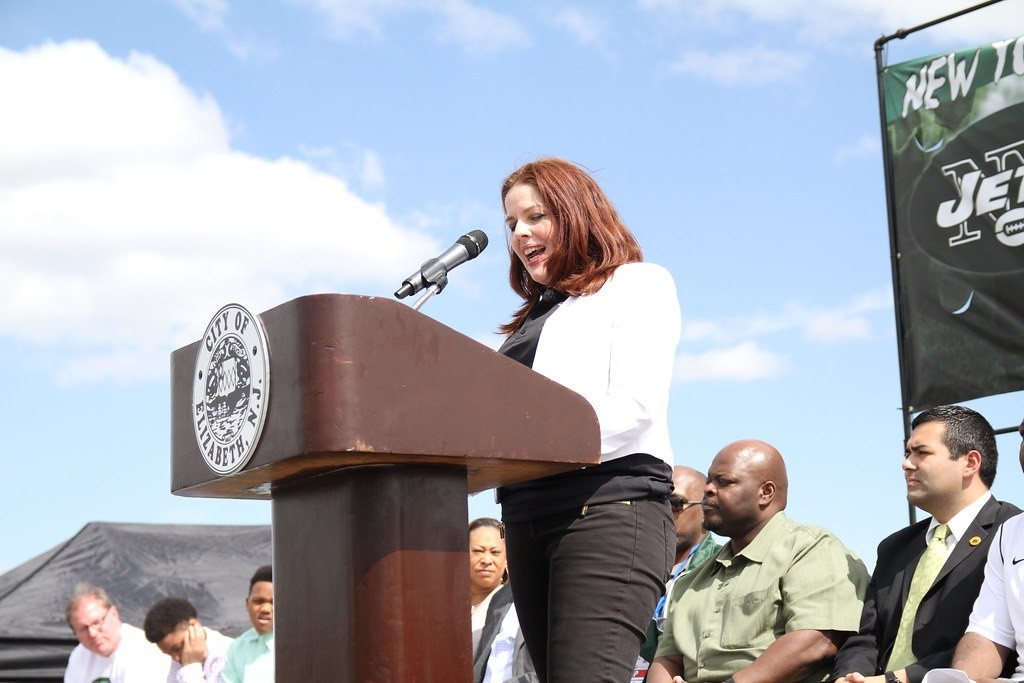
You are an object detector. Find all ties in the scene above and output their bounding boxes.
[885,524,953,675]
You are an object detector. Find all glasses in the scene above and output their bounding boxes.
[668,496,704,514]
[72,609,110,638]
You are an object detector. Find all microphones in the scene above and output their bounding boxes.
[394,230,488,299]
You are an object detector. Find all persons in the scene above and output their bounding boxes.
[475,404,1024,683]
[144,564,275,683]
[62,580,181,683]
[494,160,678,681]
